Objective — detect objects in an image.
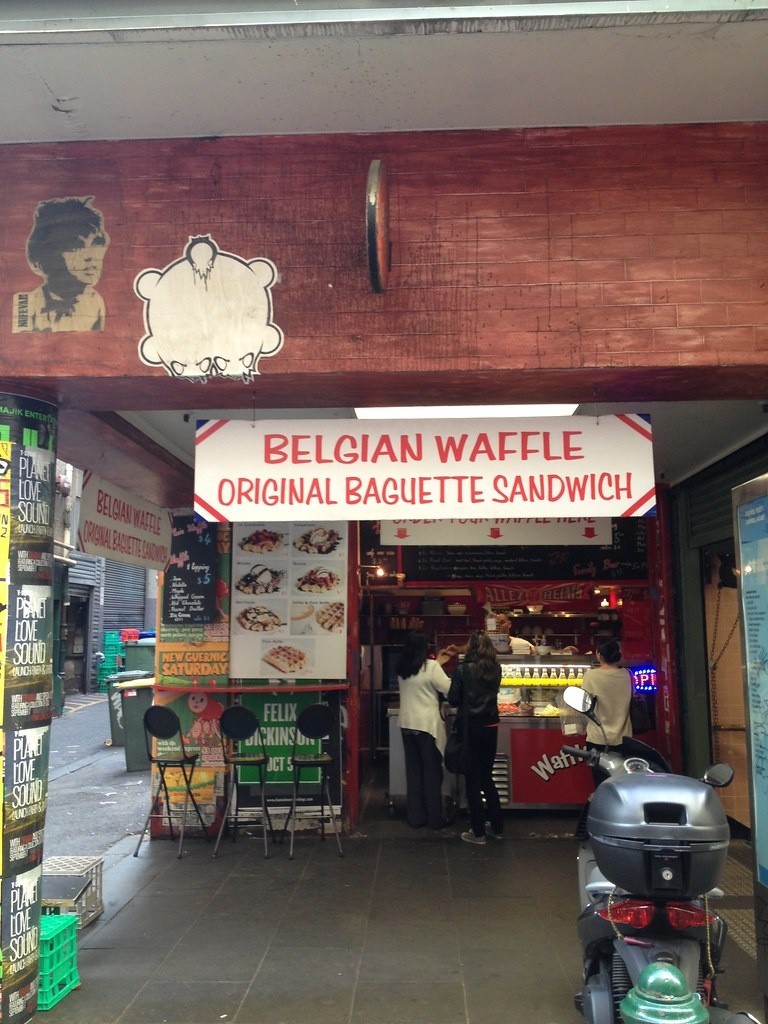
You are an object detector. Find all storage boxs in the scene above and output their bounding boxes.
[421,597,446,613]
[43,855,104,929]
[36,915,81,1011]
[95,629,155,693]
[586,774,730,900]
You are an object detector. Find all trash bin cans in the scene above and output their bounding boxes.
[105,670,155,746]
[123,637,156,671]
[115,677,156,773]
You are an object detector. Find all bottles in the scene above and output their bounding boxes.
[532,668,539,678]
[559,668,566,679]
[541,668,548,678]
[577,668,583,679]
[506,669,514,678]
[568,668,575,679]
[515,668,522,678]
[524,668,530,678]
[550,668,557,679]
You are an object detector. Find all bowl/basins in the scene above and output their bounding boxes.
[527,605,543,613]
[448,605,466,615]
[535,646,551,655]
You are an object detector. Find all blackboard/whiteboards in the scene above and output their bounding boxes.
[161,514,218,624]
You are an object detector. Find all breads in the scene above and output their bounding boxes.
[291,602,345,634]
[262,646,309,673]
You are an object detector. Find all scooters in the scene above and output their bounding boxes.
[560,685,735,1024]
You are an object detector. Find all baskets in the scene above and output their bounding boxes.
[96,631,126,692]
[120,629,139,641]
[139,630,156,639]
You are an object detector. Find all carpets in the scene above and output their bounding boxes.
[716,854,753,896]
[715,908,756,961]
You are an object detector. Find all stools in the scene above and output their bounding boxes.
[213,705,277,858]
[279,702,346,859]
[42,876,92,915]
[134,704,211,860]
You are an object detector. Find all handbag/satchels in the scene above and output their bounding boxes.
[445,731,469,775]
[626,668,651,734]
[438,652,458,702]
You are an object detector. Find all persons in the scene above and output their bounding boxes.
[447,631,503,846]
[581,636,633,790]
[397,629,451,831]
[445,611,536,657]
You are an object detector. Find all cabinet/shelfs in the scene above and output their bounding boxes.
[378,613,471,647]
[497,656,592,718]
[506,613,618,646]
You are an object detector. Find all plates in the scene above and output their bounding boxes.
[291,604,315,621]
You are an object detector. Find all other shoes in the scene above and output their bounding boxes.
[461,828,486,845]
[484,822,505,840]
[431,818,451,829]
